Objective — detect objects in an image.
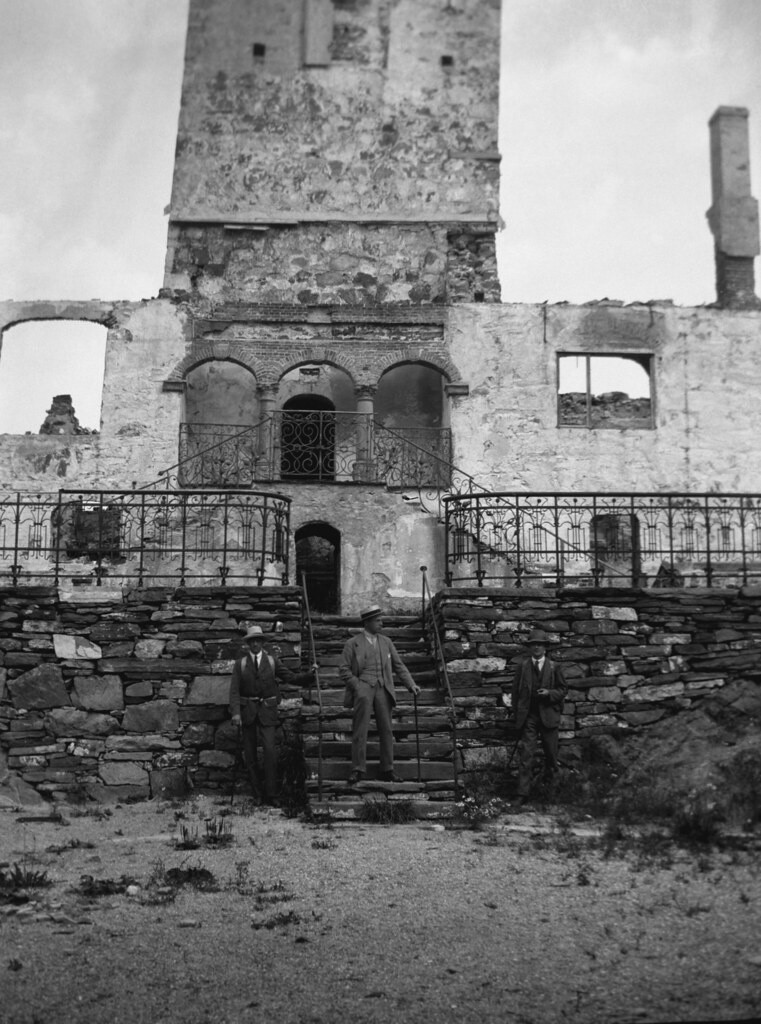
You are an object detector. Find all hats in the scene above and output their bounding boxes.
[356,605,388,624]
[242,626,269,641]
[521,630,551,647]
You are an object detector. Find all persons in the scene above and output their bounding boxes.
[228,626,317,807]
[510,631,568,809]
[340,605,422,783]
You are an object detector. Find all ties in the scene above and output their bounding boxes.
[254,655,258,671]
[534,660,540,672]
[372,634,376,648]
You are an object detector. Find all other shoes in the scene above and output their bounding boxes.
[348,771,361,782]
[377,771,404,782]
[512,796,526,807]
